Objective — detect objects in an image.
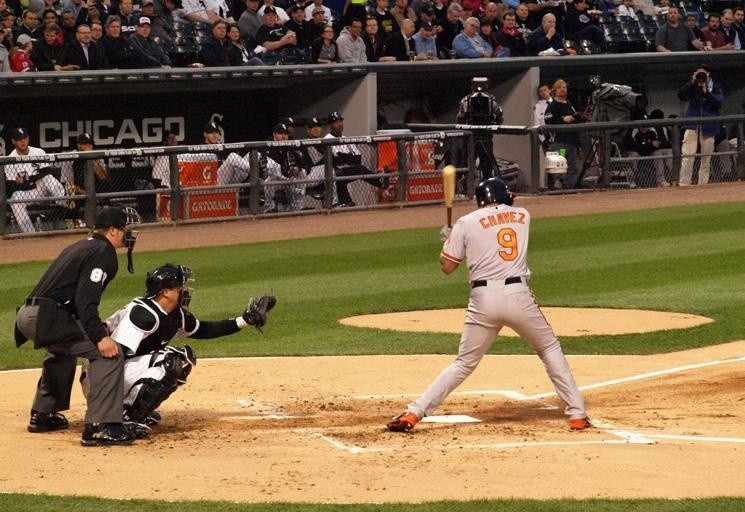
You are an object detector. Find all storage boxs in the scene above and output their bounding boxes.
[376,169,444,204]
[177,152,218,189]
[399,138,436,172]
[154,187,241,222]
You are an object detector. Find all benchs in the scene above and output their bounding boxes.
[9,162,153,221]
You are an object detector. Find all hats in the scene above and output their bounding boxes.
[311,7,325,16]
[137,16,152,27]
[328,111,344,122]
[420,20,433,30]
[16,33,37,44]
[93,204,132,229]
[281,117,296,128]
[308,117,322,128]
[202,121,221,133]
[272,123,291,134]
[142,0,154,6]
[292,3,305,13]
[78,133,94,144]
[12,127,29,141]
[264,5,277,15]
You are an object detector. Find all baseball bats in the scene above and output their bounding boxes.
[443,165,456,229]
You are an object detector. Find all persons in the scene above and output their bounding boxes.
[80,263,277,440]
[386,177,593,430]
[14,204,138,446]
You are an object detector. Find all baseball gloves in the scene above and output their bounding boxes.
[242,294,276,333]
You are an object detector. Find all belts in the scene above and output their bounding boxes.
[24,296,67,309]
[471,276,524,289]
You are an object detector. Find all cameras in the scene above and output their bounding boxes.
[695,70,707,85]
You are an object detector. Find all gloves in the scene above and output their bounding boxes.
[439,222,455,244]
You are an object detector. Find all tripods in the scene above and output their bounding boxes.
[574,134,645,189]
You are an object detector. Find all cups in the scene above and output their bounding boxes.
[449,50,456,59]
[408,50,416,62]
[699,42,705,51]
[427,50,434,61]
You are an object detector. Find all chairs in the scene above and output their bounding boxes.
[153,15,315,66]
[558,0,745,54]
[595,142,636,189]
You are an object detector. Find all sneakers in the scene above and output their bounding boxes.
[569,417,594,430]
[281,150,296,178]
[657,180,672,187]
[123,421,152,440]
[139,410,162,428]
[386,411,418,432]
[80,427,137,446]
[27,412,69,434]
[378,164,390,189]
[63,180,77,210]
[258,152,269,181]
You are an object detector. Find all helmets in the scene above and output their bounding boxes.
[144,262,188,300]
[473,177,514,209]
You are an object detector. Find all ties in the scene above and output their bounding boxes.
[546,100,550,106]
